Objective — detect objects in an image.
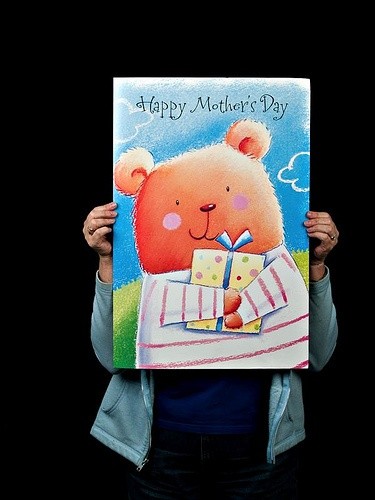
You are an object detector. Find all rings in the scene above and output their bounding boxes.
[331,235,336,239]
[87,225,95,233]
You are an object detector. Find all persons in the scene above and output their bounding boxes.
[83,202,340,500]
